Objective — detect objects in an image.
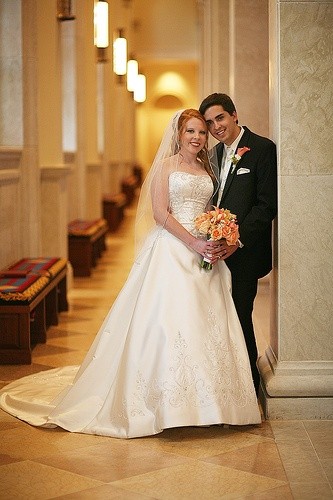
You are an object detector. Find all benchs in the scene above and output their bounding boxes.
[102,174,140,232]
[68,218,109,279]
[0,257,68,366]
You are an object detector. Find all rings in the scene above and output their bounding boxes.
[224,249,227,253]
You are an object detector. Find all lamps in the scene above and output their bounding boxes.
[93,0,147,103]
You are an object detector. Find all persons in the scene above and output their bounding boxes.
[200,93,277,400]
[0,109,262,439]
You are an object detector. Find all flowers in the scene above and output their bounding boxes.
[192,205,240,270]
[231,147,251,175]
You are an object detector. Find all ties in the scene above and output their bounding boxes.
[217,147,232,208]
